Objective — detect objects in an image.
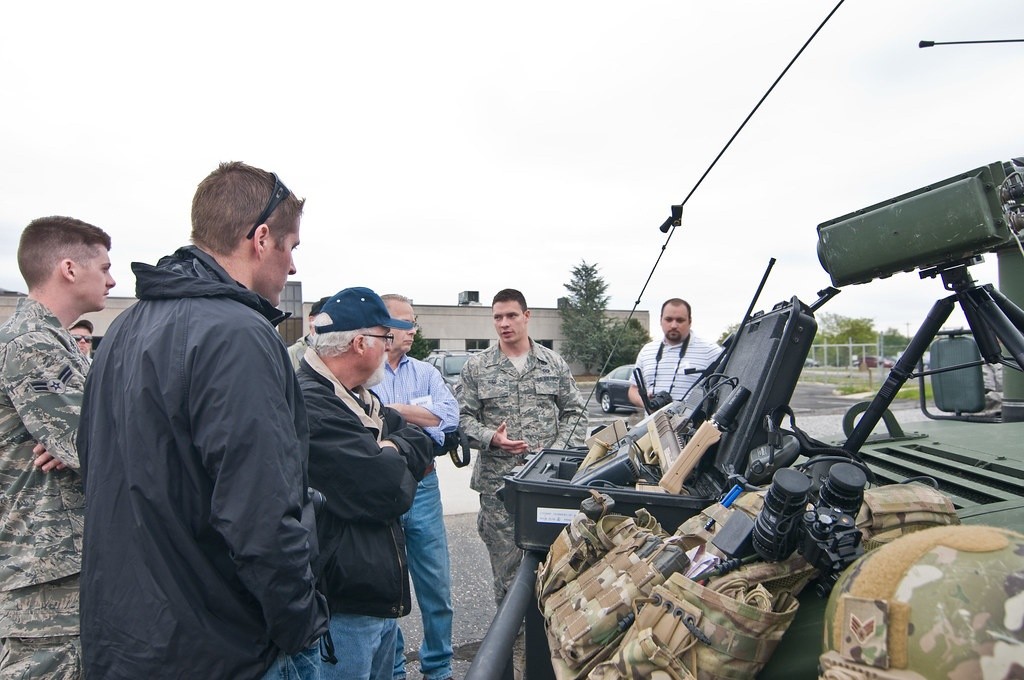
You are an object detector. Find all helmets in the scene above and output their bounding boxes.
[815,527,1024,680]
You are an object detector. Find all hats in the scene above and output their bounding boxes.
[310,297,331,315]
[315,286,414,334]
[69,318,93,334]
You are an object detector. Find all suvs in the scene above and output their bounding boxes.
[424,349,481,393]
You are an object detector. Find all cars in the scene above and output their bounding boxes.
[805,358,820,368]
[857,354,895,368]
[595,366,639,413]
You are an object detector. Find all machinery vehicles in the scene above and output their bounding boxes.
[457,154,1024,680]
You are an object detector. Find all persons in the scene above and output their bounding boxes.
[294,286,434,680]
[454,289,590,636]
[76,161,330,680]
[367,295,460,680]
[67,320,93,365]
[628,298,722,419]
[287,296,331,373]
[0,216,116,680]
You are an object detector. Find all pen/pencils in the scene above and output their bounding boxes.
[703,484,743,531]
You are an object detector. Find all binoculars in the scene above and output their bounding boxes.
[752,463,867,562]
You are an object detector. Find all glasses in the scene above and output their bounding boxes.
[73,335,93,343]
[348,333,394,346]
[246,171,291,239]
[411,323,419,332]
